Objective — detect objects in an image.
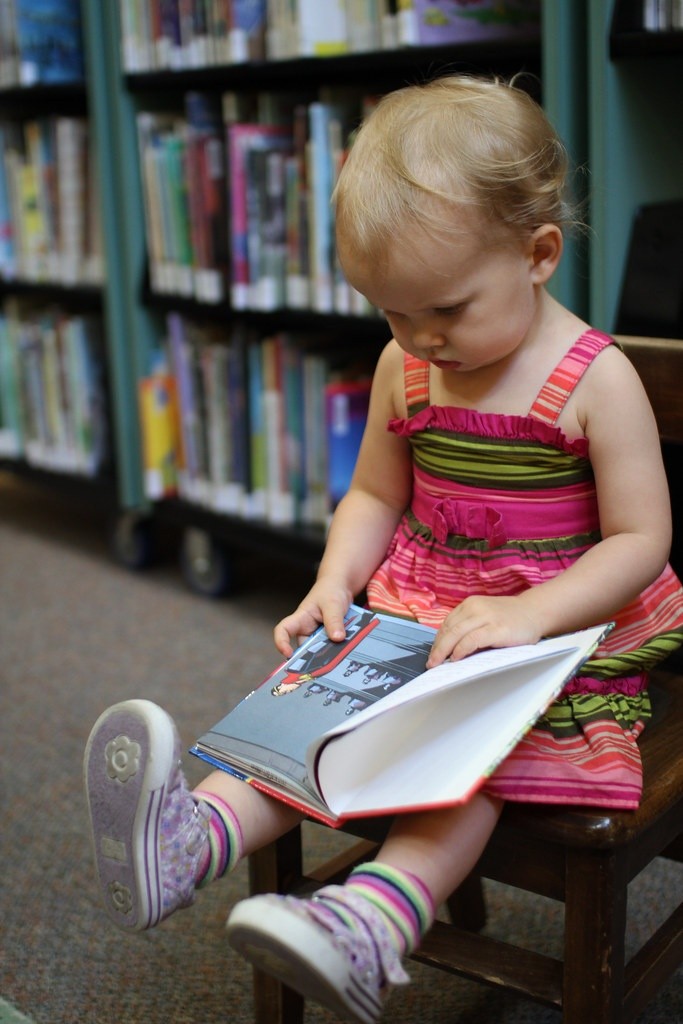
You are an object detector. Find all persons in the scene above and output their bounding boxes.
[84,75,683,1024]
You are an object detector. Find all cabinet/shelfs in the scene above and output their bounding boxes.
[1,0,682,674]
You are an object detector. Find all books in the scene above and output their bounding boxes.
[188,603,616,832]
[0,0,516,544]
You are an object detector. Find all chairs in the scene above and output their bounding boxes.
[247,336,683,1024]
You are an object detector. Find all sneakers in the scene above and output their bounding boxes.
[224,893,412,1024]
[84,701,211,936]
